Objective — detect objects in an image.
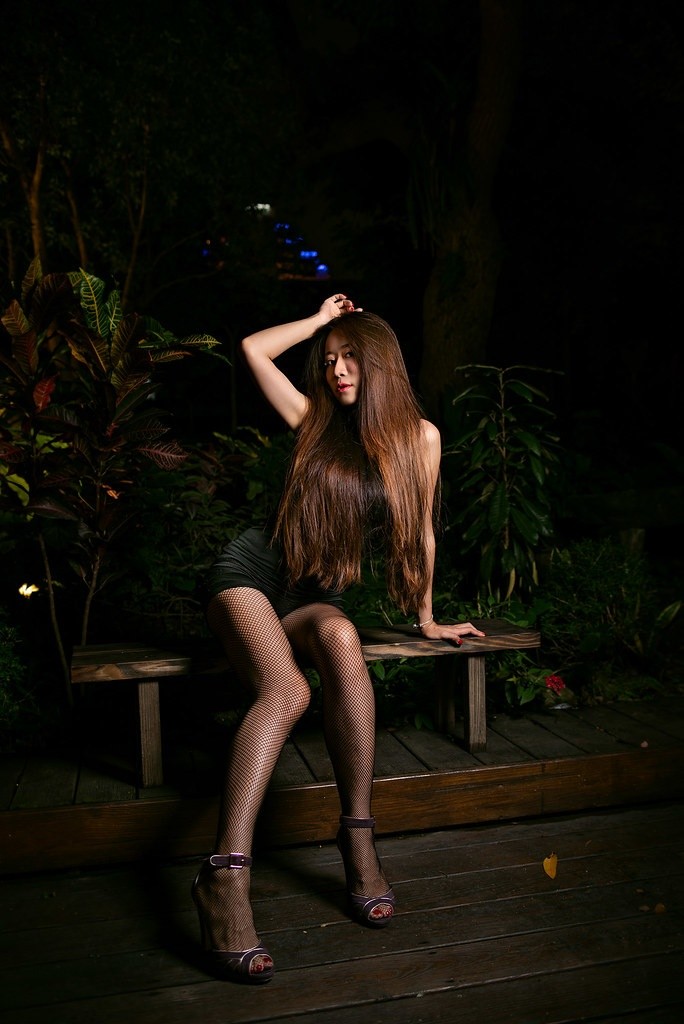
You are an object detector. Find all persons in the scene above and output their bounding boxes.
[190,294,486,981]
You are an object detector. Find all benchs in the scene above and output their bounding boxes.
[71,620,540,789]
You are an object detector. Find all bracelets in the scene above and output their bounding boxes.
[413,614,433,629]
[423,621,434,634]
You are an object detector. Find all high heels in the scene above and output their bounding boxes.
[191,852,273,984]
[333,814,395,928]
